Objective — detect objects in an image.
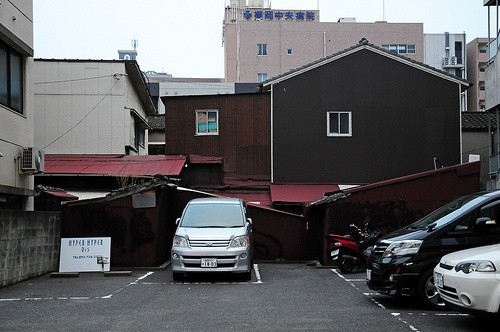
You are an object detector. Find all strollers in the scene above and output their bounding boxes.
[350,224,375,273]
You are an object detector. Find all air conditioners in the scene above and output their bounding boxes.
[21,145,45,174]
[444,57,457,65]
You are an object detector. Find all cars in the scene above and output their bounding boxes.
[434,243,500,314]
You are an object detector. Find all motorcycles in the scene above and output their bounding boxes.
[327,222,382,274]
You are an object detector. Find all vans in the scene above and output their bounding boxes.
[366,189,499,312]
[171,197,253,282]
